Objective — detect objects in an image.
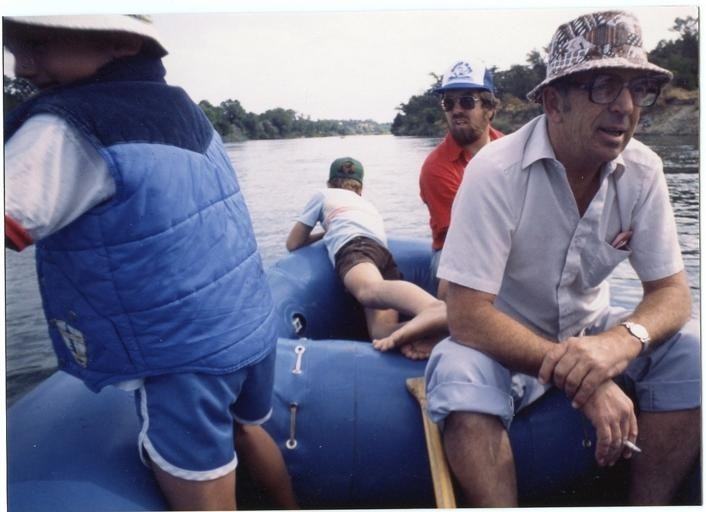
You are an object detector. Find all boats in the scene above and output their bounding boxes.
[6,235,701,512]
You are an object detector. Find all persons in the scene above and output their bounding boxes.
[423,10,703,506]
[287,157,448,362]
[1,0,298,512]
[419,58,507,300]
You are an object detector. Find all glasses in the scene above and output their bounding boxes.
[440,96,481,111]
[565,72,661,107]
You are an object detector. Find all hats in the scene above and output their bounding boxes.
[432,60,495,94]
[3,15,168,58]
[526,10,674,104]
[329,157,364,184]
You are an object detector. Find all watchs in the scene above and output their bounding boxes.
[619,321,650,352]
